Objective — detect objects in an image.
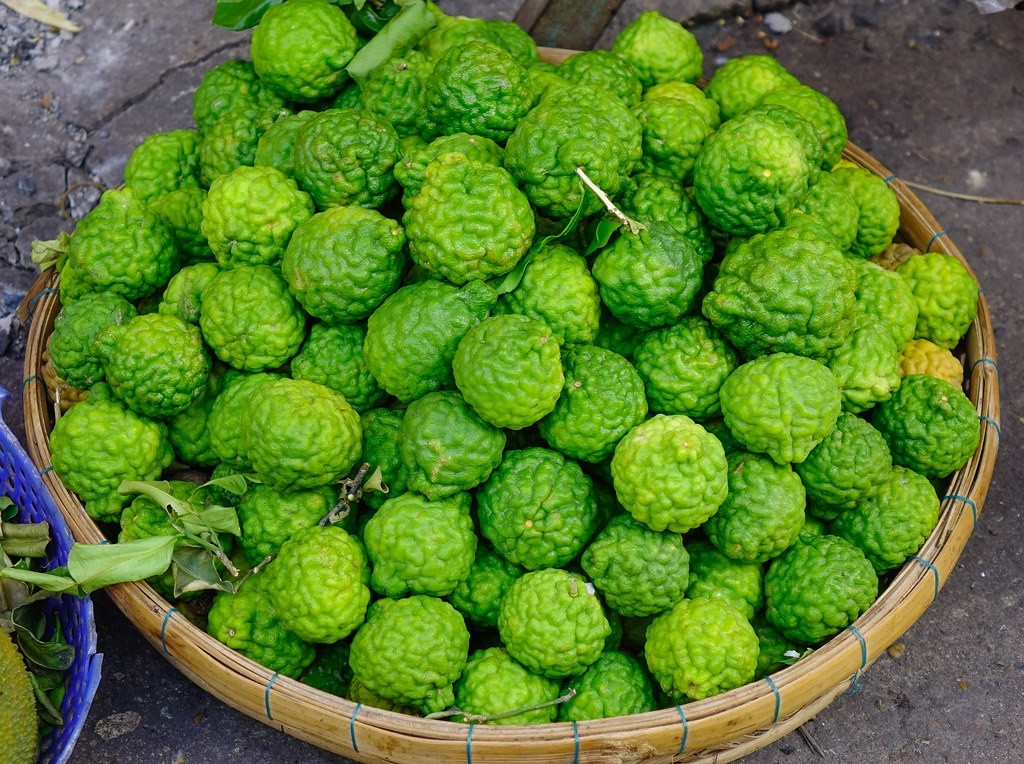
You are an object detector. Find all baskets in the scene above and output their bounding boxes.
[0,414,106,764]
[21,43,998,761]
